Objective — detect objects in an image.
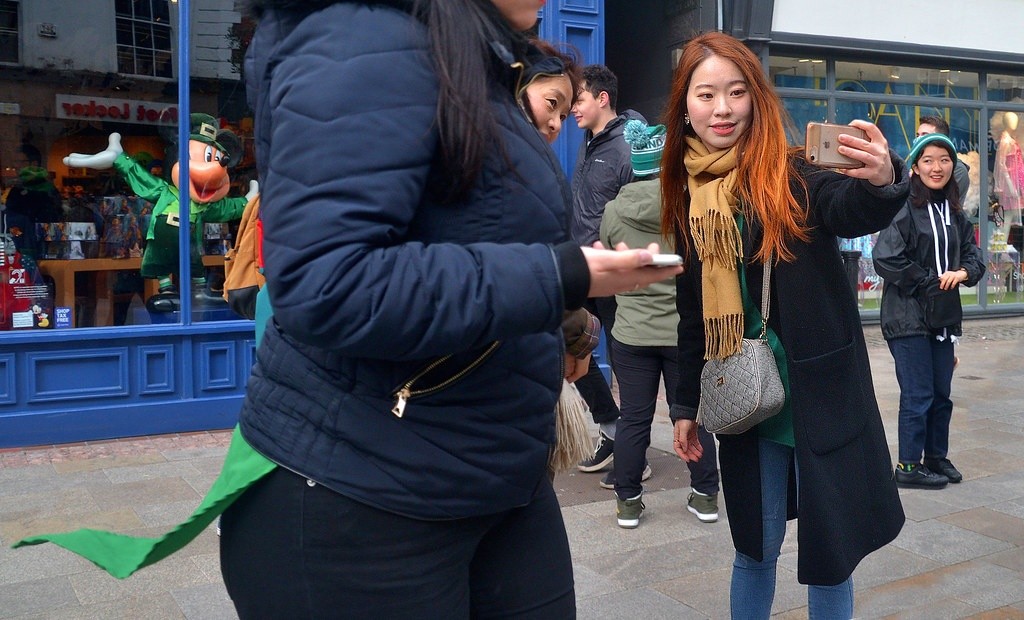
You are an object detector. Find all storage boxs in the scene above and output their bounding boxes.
[35,196,154,260]
[204,223,232,256]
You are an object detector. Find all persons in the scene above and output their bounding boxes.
[514,37,600,385]
[913,115,970,211]
[659,32,911,620]
[597,120,721,529]
[870,133,986,490]
[10,0,685,619]
[561,63,654,489]
[991,111,1024,242]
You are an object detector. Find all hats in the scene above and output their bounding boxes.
[625,119,667,177]
[906,133,959,172]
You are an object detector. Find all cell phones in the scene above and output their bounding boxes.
[582,247,682,267]
[806,121,870,168]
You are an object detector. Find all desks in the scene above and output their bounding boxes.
[36,255,224,328]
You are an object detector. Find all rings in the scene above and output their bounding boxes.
[633,285,640,291]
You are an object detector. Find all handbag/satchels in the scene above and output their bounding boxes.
[695,334,786,434]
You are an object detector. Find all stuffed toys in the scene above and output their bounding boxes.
[60,112,259,311]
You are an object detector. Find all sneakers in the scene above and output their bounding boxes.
[687,488,719,523]
[923,455,963,482]
[614,490,645,528]
[577,427,614,471]
[599,458,651,488]
[894,462,949,489]
